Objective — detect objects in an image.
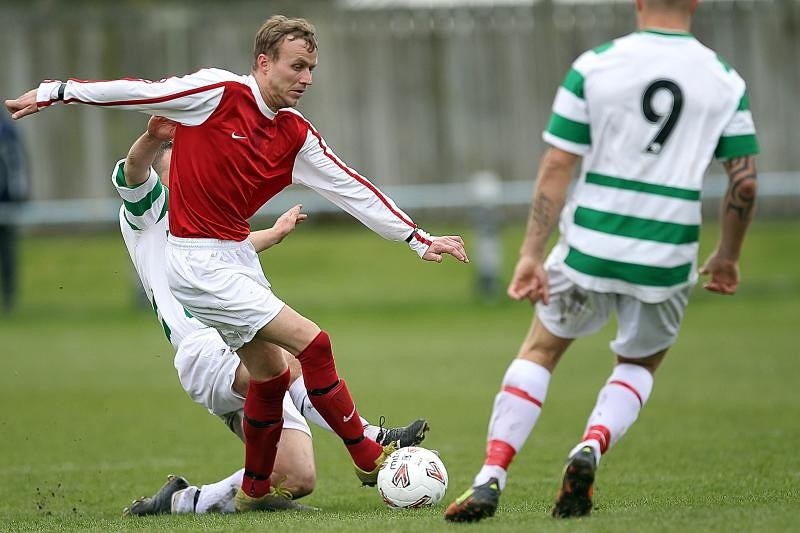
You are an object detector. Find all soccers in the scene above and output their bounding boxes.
[377,446,449,509]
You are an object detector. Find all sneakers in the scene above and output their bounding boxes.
[123,475,190,515]
[351,448,441,486]
[381,419,430,447]
[235,486,323,513]
[442,478,501,521]
[549,446,597,518]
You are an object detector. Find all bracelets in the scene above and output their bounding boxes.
[57,82,67,103]
[405,227,420,243]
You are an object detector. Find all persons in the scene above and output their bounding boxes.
[5,14,470,514]
[445,0,761,522]
[111,116,431,515]
